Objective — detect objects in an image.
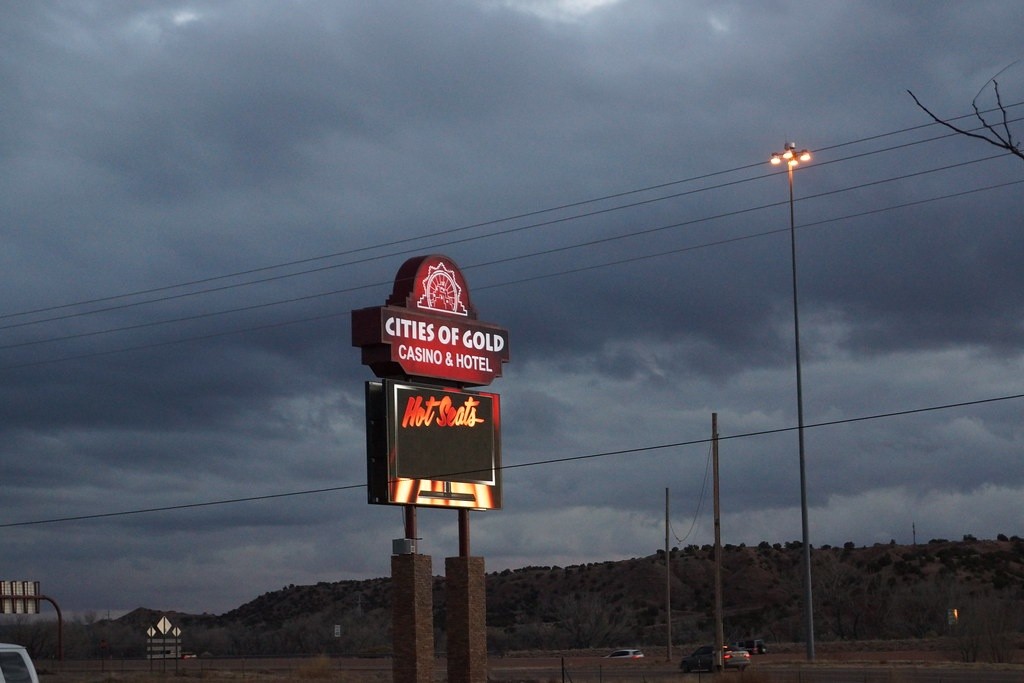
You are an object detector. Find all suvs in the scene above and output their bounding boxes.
[730,639,767,655]
[602,649,644,659]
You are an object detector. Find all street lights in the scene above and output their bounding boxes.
[771,141,816,660]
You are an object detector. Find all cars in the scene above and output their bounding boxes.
[679,644,751,673]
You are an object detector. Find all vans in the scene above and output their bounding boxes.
[0,643,40,683]
